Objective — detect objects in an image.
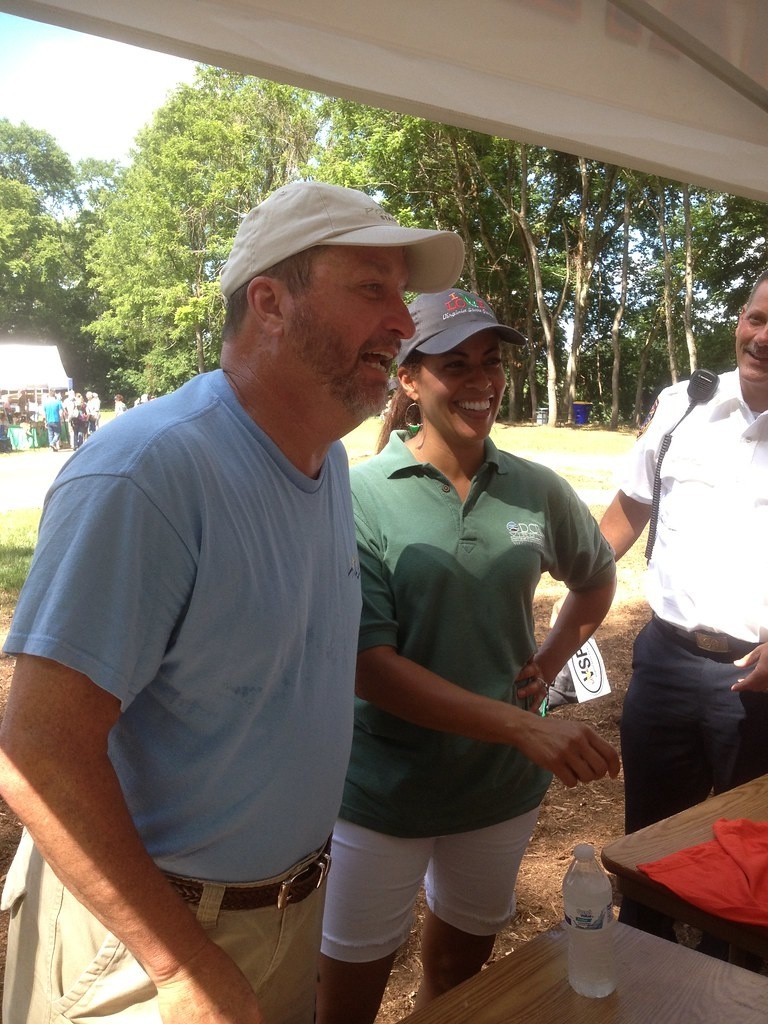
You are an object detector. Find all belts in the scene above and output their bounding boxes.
[649,612,758,654]
[166,832,335,910]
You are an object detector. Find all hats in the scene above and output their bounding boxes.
[395,290,524,365]
[220,182,464,295]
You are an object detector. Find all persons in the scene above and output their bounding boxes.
[0,180,465,1022]
[319,288,617,1024]
[594,274,767,832]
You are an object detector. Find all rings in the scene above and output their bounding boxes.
[536,678,548,689]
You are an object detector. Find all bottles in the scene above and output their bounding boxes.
[565,842,620,1000]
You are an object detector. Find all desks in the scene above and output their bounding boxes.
[602,772,768,976]
[393,913,767,1024]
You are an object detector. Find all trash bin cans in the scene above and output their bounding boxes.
[536,408,548,423]
[573,401,593,424]
